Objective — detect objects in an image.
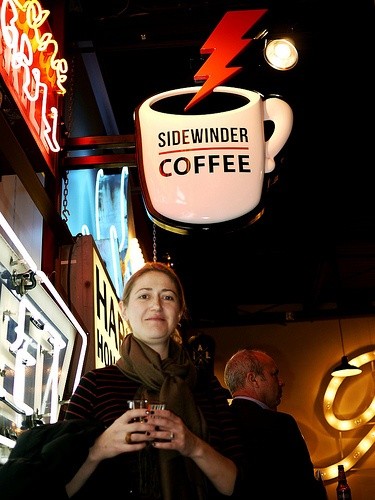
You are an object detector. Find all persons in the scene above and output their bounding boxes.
[63,262,255,500]
[200,349,327,500]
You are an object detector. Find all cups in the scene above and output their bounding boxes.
[127,399,167,446]
[136,85,293,227]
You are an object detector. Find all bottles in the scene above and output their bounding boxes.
[315,470,322,481]
[336,465,351,500]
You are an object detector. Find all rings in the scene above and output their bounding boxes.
[171,432,176,439]
[125,432,131,443]
